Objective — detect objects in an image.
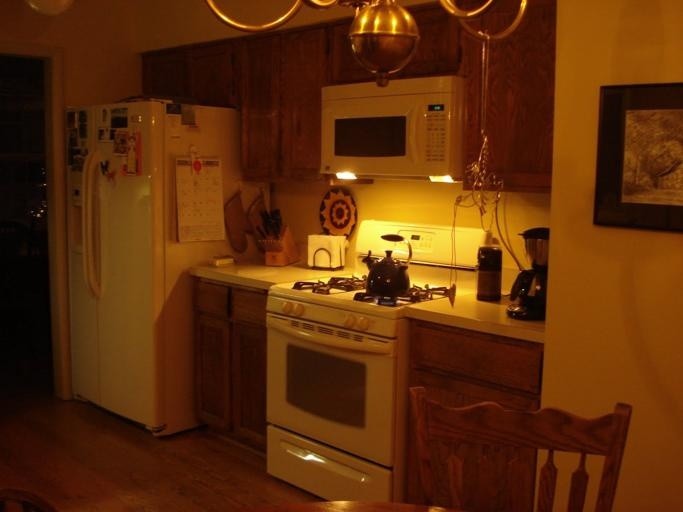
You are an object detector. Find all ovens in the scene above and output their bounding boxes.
[263,310,414,506]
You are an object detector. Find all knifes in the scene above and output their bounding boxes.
[255,207,284,241]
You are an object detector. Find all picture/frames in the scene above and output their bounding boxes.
[593,82,682,236]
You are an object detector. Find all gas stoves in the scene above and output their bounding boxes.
[265,269,451,339]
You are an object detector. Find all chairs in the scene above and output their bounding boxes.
[405,384,634,512]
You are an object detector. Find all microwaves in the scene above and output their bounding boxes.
[319,74,467,184]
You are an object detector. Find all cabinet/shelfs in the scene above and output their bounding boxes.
[406,320,545,511]
[193,276,266,454]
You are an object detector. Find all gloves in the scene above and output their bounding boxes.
[223,191,255,254]
[247,194,273,253]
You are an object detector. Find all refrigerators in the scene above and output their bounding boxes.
[59,96,248,444]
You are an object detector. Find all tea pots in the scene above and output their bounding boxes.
[360,233,414,299]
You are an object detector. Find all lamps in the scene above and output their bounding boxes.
[205,0,530,86]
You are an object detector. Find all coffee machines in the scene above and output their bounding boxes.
[506,226,550,322]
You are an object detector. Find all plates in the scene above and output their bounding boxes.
[317,188,357,240]
